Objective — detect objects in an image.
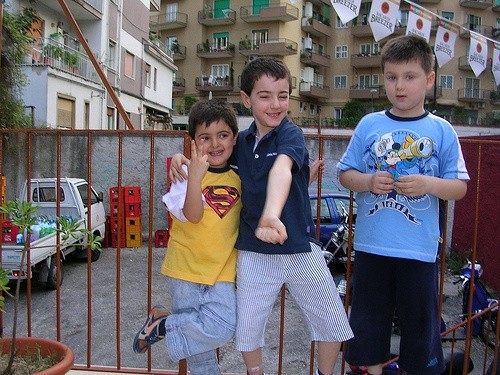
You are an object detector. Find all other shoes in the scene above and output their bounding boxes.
[315,368,335,375]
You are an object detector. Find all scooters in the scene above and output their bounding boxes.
[390,258,499,351]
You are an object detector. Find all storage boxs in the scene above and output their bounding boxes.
[155,229,168,247]
[2,219,18,242]
[110,186,141,247]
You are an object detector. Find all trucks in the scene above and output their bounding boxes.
[0,176,108,291]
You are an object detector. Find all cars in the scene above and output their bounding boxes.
[308,189,358,274]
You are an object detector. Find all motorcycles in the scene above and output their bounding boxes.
[322,200,357,274]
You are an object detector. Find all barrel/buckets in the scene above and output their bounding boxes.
[30,225,40,243]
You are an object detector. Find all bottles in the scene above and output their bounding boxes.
[47,218,56,229]
[17,234,23,243]
[38,216,48,239]
[61,215,73,223]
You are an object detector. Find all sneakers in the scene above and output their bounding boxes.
[133,305,173,355]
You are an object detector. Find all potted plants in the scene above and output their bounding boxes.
[0,200,101,375]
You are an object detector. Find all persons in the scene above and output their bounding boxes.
[169,57,354,375]
[335,37,470,375]
[134,96,325,375]
